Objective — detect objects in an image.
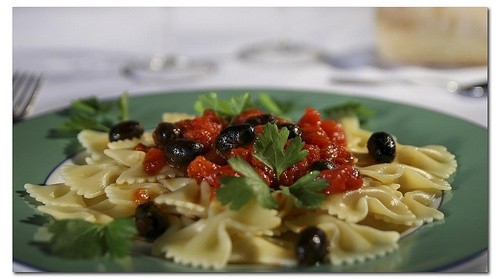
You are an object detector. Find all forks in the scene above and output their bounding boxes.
[13,69,43,123]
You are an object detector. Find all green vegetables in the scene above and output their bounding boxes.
[24,91,458,272]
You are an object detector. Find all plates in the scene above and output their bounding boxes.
[14,88,487,273]
